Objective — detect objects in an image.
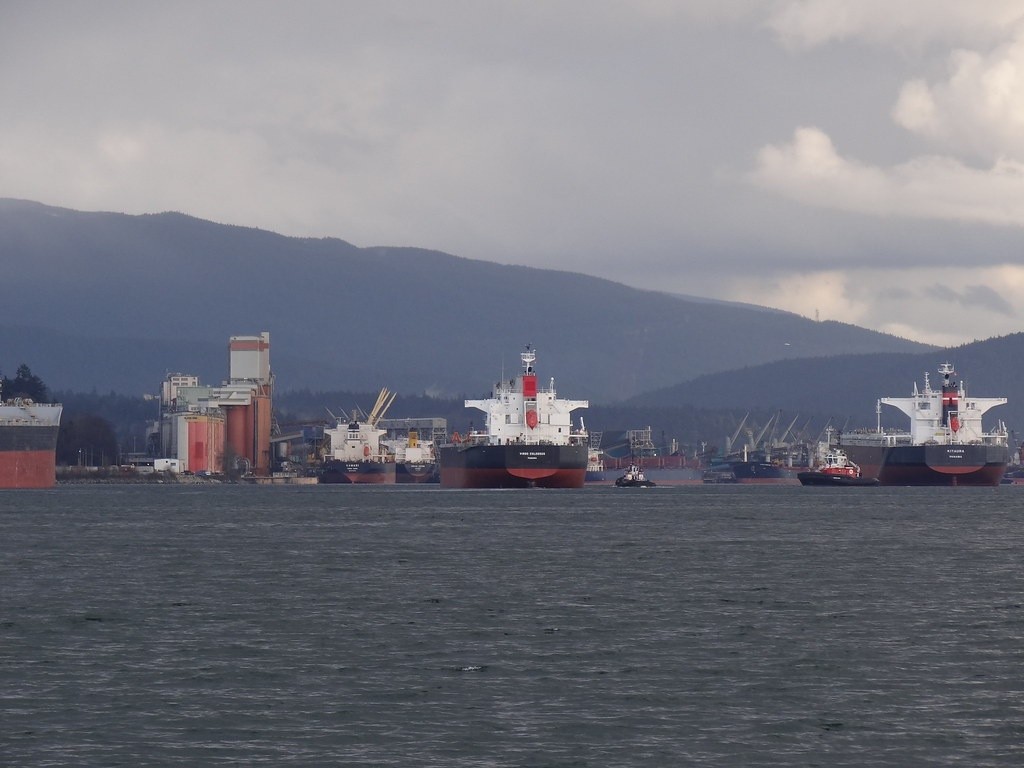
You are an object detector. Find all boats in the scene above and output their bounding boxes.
[1000,430,1023,485]
[710,424,852,485]
[584,446,606,482]
[797,449,878,485]
[616,463,656,488]
[840,360,1009,485]
[702,470,738,484]
[380,432,438,483]
[315,421,397,484]
[439,342,589,487]
